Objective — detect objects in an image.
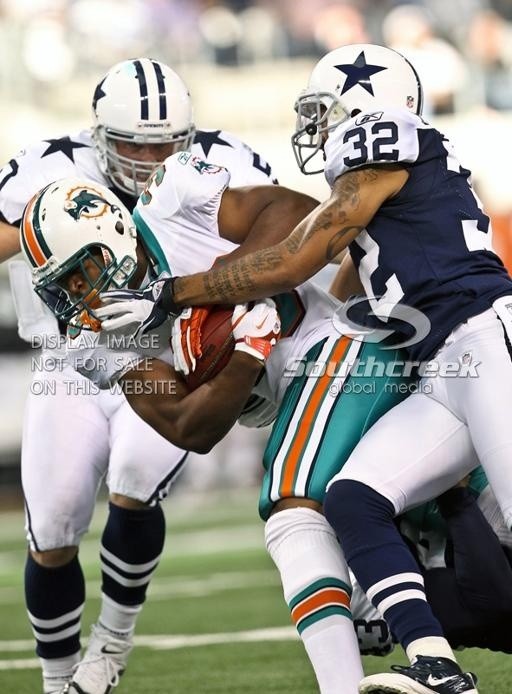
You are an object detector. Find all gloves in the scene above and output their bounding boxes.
[170,305,209,376]
[231,296,282,364]
[93,277,180,342]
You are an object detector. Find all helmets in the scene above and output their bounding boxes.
[18,176,138,332]
[92,57,195,198]
[307,42,424,137]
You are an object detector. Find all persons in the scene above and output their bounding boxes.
[0,53,286,694]
[15,147,512,694]
[345,463,512,658]
[2,1,512,491]
[90,38,512,694]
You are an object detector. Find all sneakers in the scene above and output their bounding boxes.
[357,655,481,694]
[60,622,135,694]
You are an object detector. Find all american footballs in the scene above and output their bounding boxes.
[183,305,235,391]
